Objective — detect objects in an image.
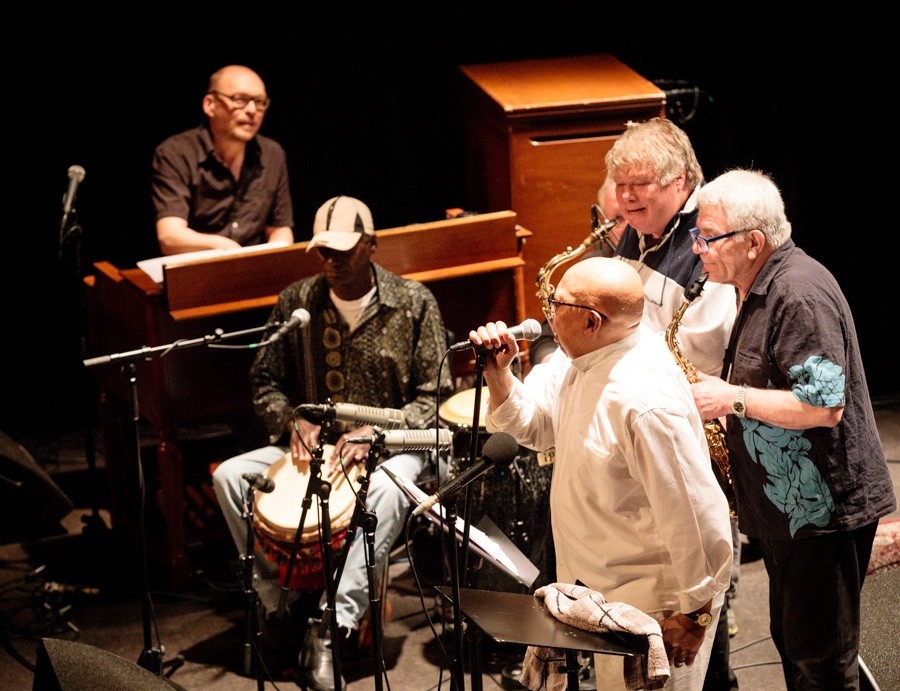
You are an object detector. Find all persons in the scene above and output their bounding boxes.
[469,116,896,691]
[151,66,453,691]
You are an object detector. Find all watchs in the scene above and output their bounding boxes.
[684,610,713,626]
[732,386,747,420]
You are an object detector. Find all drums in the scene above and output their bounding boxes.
[436,383,552,595]
[251,445,367,592]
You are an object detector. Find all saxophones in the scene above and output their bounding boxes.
[534,219,617,336]
[665,272,737,516]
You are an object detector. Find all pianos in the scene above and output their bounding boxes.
[82,206,533,570]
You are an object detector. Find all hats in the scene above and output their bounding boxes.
[304,195,375,254]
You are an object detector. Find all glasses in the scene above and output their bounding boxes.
[686,227,745,252]
[207,90,271,110]
[548,293,608,320]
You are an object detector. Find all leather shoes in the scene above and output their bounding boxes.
[297,624,346,691]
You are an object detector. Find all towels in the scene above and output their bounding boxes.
[518,583,671,691]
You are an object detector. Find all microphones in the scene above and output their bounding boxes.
[349,428,452,451]
[590,206,603,250]
[62,165,86,224]
[303,402,405,430]
[243,472,275,493]
[450,319,542,351]
[269,308,311,343]
[411,433,519,516]
